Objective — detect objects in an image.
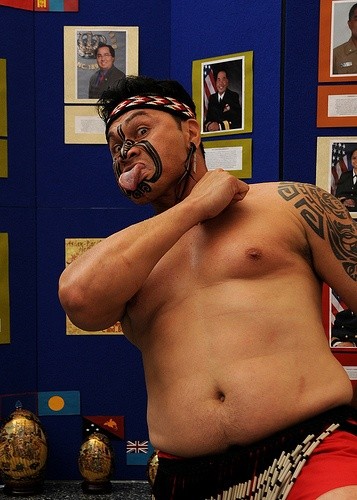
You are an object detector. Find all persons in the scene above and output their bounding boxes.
[333,3,356,77]
[202,69,242,133]
[57,75,357,499]
[89,42,126,99]
[331,306,356,349]
[333,149,357,212]
